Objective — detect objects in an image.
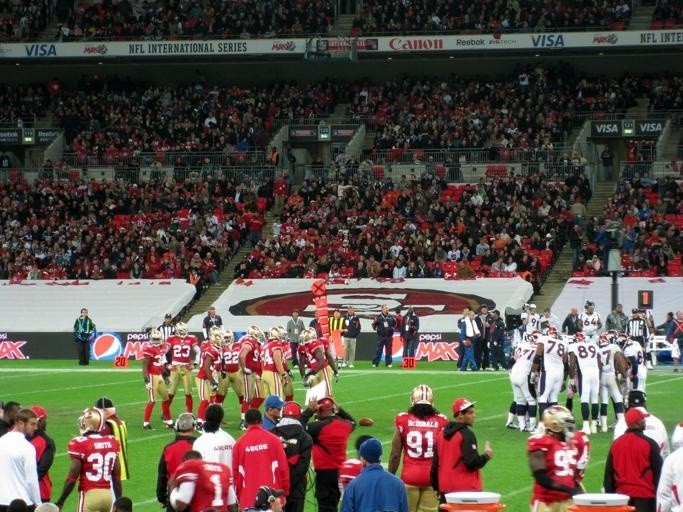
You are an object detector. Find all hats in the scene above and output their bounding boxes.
[165,314,171,319]
[95,397,113,408]
[31,406,47,421]
[255,488,283,504]
[452,397,477,414]
[206,395,285,422]
[359,438,382,463]
[625,390,650,426]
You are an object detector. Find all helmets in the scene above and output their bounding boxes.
[632,308,638,313]
[599,329,630,346]
[410,384,434,406]
[297,327,317,345]
[79,406,105,436]
[572,332,585,343]
[208,325,234,348]
[541,405,575,433]
[247,325,288,339]
[546,327,557,338]
[149,322,189,347]
[282,399,333,417]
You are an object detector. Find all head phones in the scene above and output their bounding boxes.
[624,388,647,409]
[255,485,275,503]
[280,401,304,419]
[173,412,197,431]
[77,406,104,433]
[317,398,337,414]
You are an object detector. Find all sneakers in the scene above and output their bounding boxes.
[335,362,356,370]
[577,421,617,435]
[506,422,536,435]
[372,364,392,368]
[142,416,175,429]
[239,422,248,431]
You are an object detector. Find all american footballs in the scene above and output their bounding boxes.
[358,418,374,426]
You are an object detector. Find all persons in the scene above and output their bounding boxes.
[0,1,683,512]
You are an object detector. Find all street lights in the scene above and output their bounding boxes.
[606,247,622,309]
